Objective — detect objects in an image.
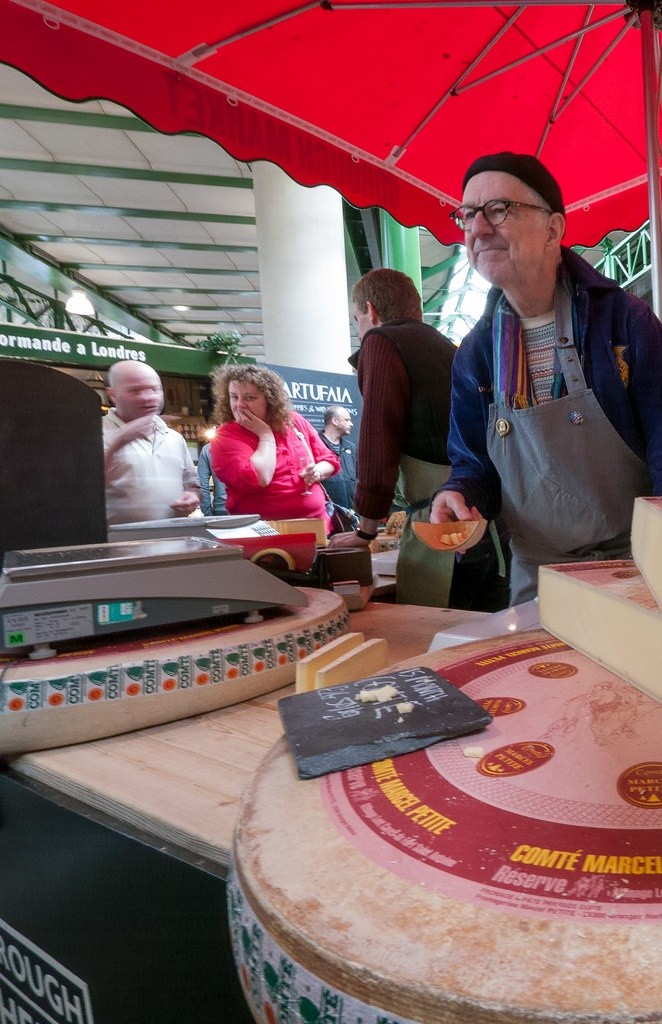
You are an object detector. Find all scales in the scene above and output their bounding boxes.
[107,513,280,541]
[0,535,311,662]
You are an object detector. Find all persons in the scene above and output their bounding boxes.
[427,149,662,611]
[315,404,358,534]
[328,266,513,615]
[198,438,228,516]
[212,361,342,548]
[101,360,202,526]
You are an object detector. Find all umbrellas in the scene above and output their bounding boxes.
[1,0,662,326]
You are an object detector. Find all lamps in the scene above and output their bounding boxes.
[65,287,95,316]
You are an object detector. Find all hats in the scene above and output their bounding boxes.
[461,151,567,241]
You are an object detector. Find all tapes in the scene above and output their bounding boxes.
[250,547,296,571]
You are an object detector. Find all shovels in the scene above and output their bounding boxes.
[412,519,487,552]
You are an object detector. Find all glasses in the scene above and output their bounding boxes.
[449,198,552,231]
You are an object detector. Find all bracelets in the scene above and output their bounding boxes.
[356,527,378,540]
[316,469,323,481]
